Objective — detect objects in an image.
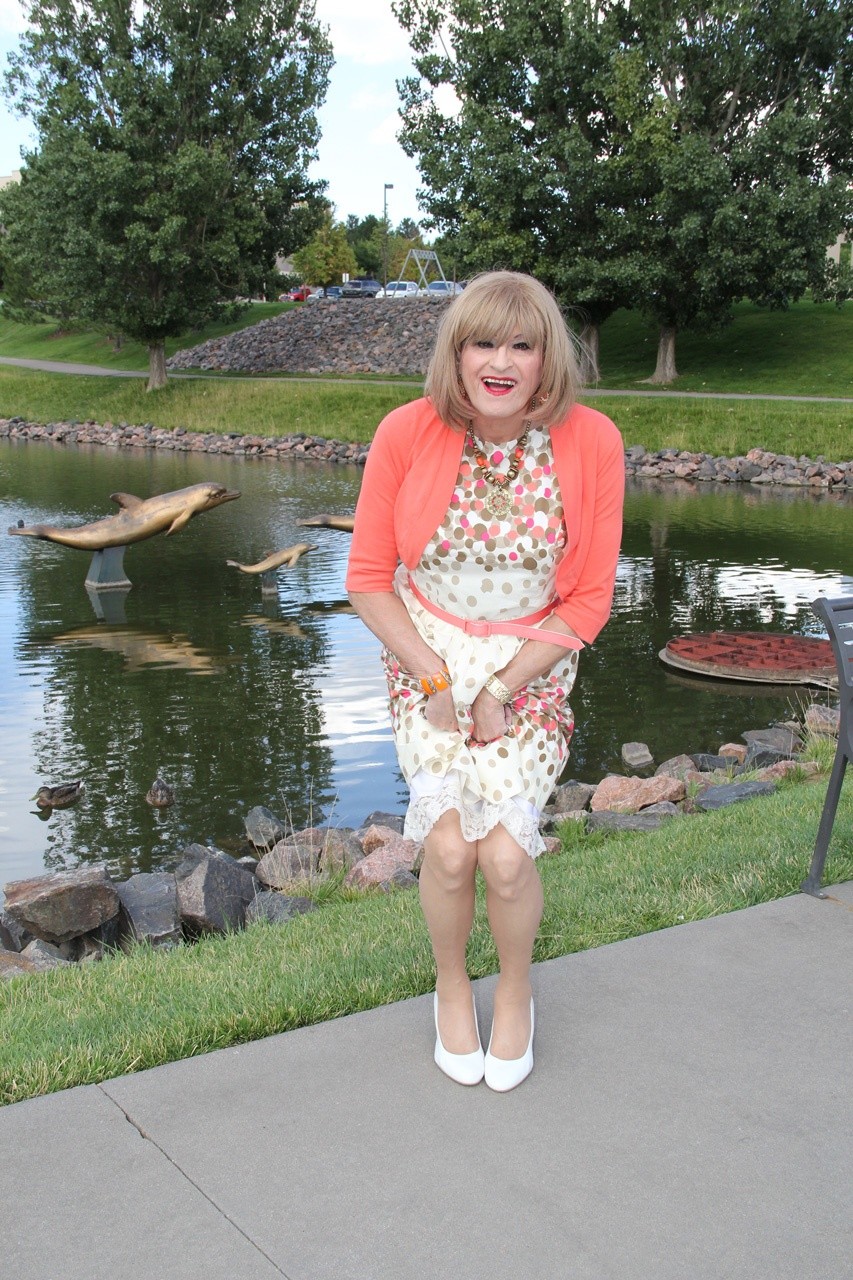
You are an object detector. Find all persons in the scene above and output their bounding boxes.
[346,270,625,1092]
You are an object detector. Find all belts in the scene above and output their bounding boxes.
[408,573,585,652]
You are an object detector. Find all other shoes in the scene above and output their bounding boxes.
[433,991,485,1086]
[484,995,535,1093]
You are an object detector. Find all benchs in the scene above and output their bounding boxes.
[801,596,853,900]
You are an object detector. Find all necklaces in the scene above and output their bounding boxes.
[467,395,536,488]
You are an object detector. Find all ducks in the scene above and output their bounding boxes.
[28,780,81,805]
[146,778,174,807]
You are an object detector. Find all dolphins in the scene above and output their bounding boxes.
[226,541,319,575]
[9,481,242,550]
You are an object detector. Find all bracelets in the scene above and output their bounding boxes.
[484,676,512,708]
[418,660,451,697]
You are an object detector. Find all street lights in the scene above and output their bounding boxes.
[384,182,394,297]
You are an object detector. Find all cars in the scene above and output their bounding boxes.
[375,281,421,299]
[417,280,464,297]
[338,280,384,299]
[306,288,342,301]
[278,287,311,303]
